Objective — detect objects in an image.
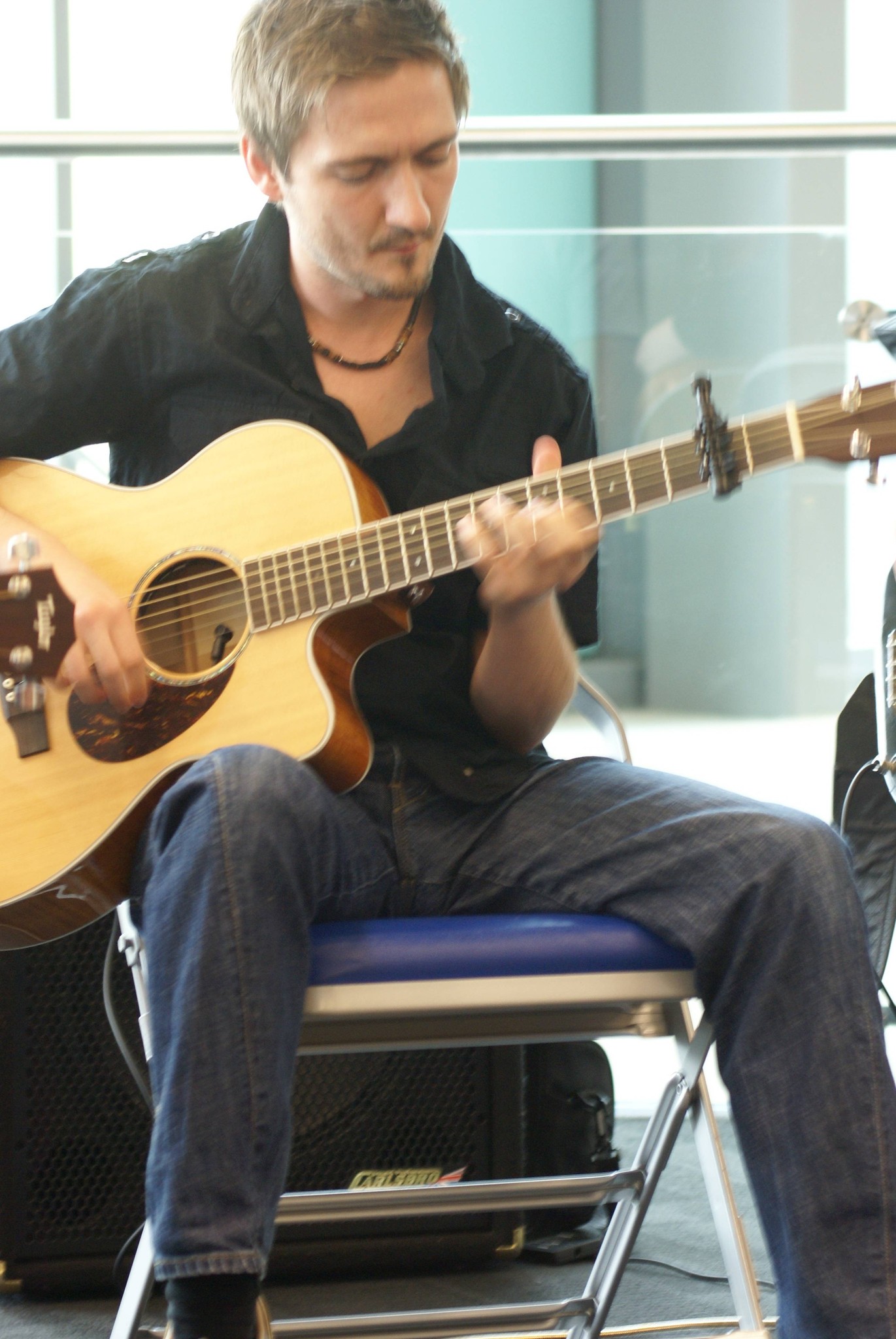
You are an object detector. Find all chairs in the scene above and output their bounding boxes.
[108,678,772,1339]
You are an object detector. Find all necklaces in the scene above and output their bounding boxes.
[308,287,423,370]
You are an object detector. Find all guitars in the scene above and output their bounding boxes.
[0,363,896,967]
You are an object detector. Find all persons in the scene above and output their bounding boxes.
[0,0,895,1339]
